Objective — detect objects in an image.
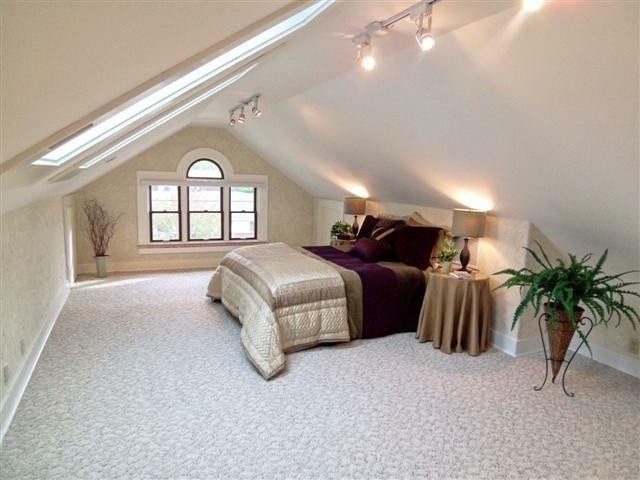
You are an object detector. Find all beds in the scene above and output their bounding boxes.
[219,244,437,354]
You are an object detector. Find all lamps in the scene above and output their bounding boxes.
[229,94,262,126]
[451,208,486,274]
[343,197,366,240]
[352,0,440,70]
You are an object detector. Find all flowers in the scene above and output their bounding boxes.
[437,237,461,263]
[330,219,353,239]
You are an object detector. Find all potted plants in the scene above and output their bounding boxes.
[491,237,640,397]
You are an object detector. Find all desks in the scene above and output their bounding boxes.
[331,238,355,245]
[429,271,489,353]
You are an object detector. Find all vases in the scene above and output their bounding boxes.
[338,233,344,239]
[442,262,451,273]
[95,255,108,278]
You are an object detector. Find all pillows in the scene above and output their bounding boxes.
[351,212,447,271]
